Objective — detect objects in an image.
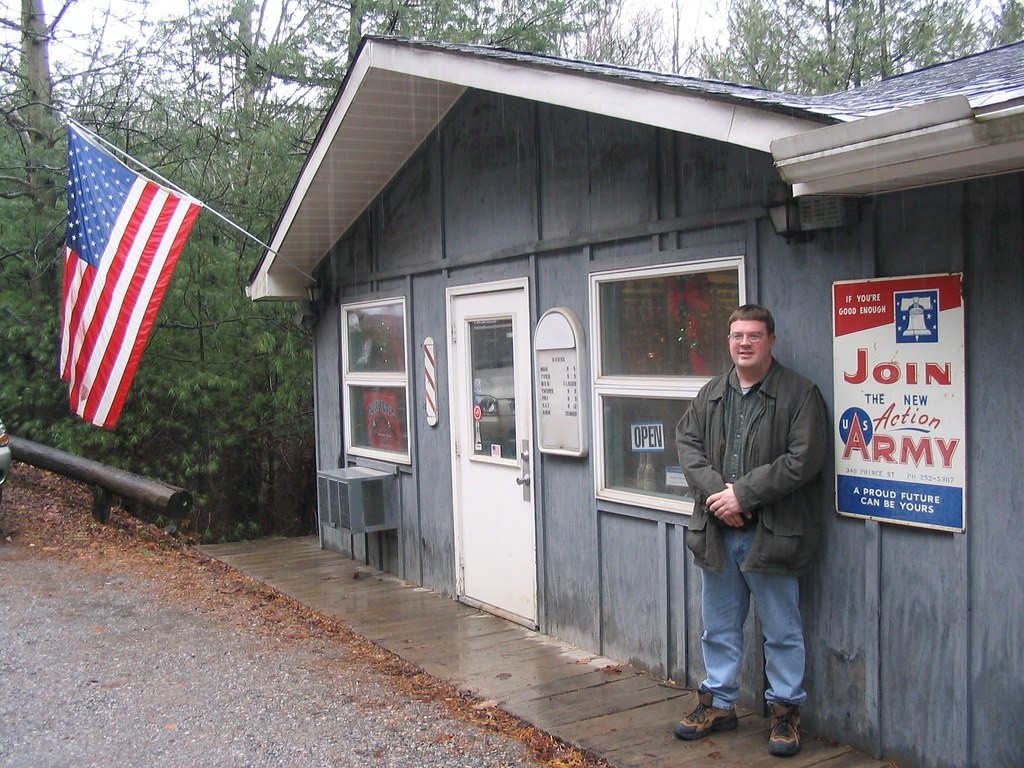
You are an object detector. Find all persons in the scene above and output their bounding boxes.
[672,303,829,757]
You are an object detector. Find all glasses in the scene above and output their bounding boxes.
[727,332,768,343]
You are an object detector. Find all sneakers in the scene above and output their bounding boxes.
[674,689,738,739]
[768,701,801,755]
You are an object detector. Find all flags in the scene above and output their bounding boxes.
[54,120,203,431]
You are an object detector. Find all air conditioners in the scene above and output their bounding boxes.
[317,467,399,535]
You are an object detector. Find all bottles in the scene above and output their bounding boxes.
[636,452,645,490]
[644,453,655,492]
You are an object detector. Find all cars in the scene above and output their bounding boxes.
[472,364,515,439]
